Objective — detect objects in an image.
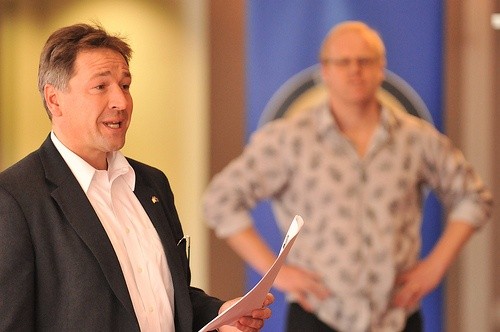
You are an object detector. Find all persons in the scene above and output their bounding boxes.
[201,19,494,332]
[2,22,276,332]
